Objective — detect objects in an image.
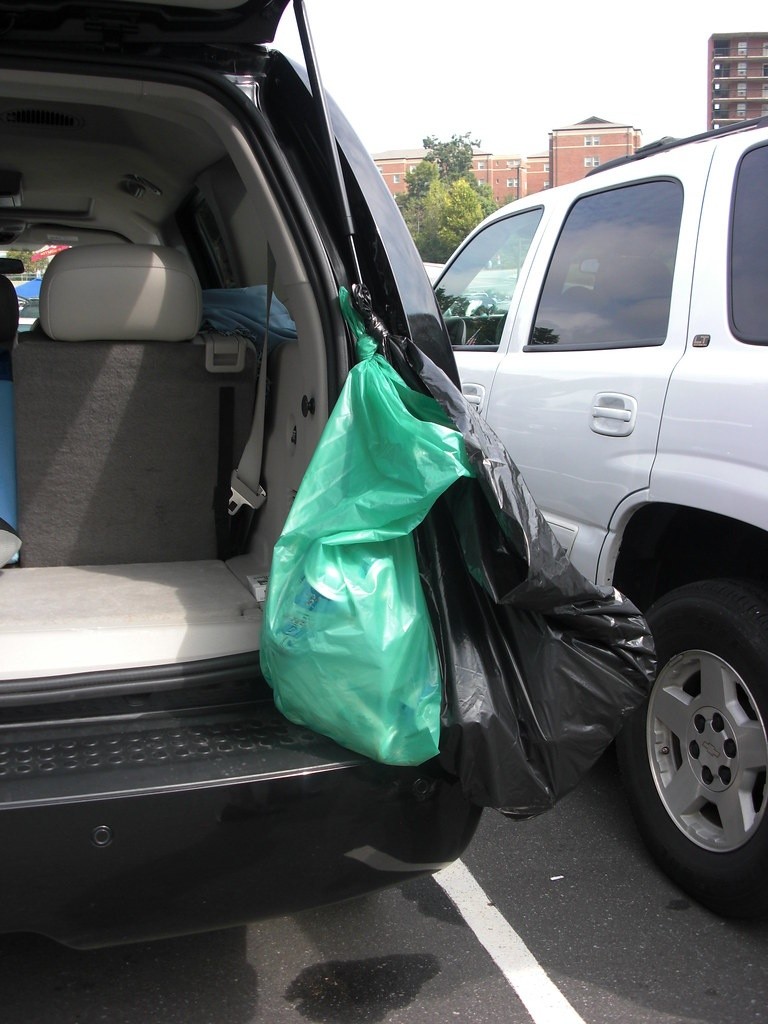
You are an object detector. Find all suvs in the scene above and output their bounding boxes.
[0,1,486,954]
[432,112,768,911]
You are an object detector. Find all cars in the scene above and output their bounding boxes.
[6,272,42,322]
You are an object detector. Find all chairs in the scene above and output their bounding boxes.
[11,241,260,564]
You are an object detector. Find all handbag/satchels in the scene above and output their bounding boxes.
[396,346,657,821]
[256,352,477,764]
